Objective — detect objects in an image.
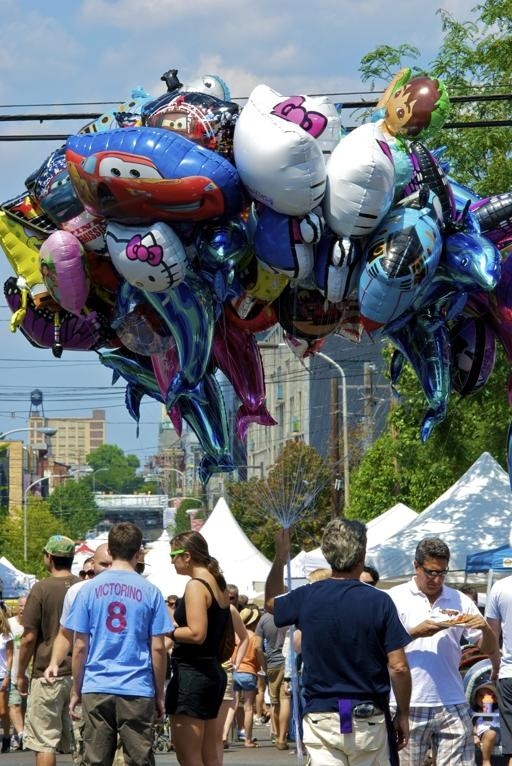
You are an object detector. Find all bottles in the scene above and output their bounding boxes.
[481,694,493,713]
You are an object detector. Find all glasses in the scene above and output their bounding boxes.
[416,559,450,577]
[95,559,113,569]
[78,569,96,580]
[169,549,186,561]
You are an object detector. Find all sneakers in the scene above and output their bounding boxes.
[223,711,290,751]
[1,732,30,753]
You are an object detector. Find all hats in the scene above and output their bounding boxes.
[137,549,153,569]
[239,607,259,627]
[43,534,76,559]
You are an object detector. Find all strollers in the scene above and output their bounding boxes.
[154,717,174,752]
[457,643,505,766]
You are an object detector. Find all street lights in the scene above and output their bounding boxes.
[141,466,187,498]
[256,337,350,524]
[0,425,61,451]
[92,467,111,495]
[69,467,94,483]
[22,473,78,568]
